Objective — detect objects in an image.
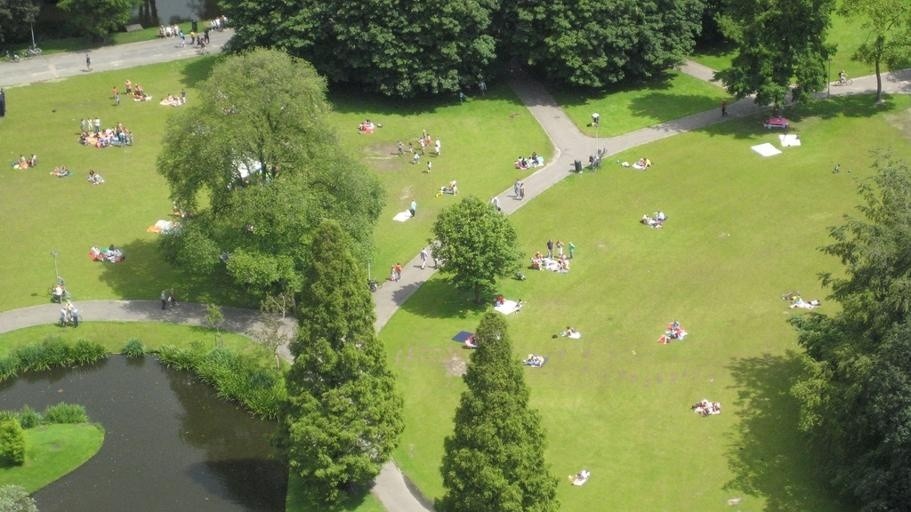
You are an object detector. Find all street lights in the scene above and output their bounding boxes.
[364,256,373,284]
[50,250,58,284]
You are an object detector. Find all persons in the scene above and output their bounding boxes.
[691,399,721,416]
[657,320,687,345]
[790,295,821,311]
[838,70,847,84]
[396,129,441,174]
[107,244,126,264]
[12,153,37,170]
[641,210,666,229]
[88,168,104,185]
[523,352,545,368]
[52,275,79,328]
[514,181,526,200]
[172,205,194,218]
[421,242,438,270]
[80,116,134,149]
[464,335,477,348]
[720,100,728,117]
[160,288,180,310]
[531,238,575,273]
[360,119,375,134]
[391,262,402,283]
[514,151,544,170]
[85,54,91,71]
[410,199,416,217]
[159,14,228,55]
[440,179,458,196]
[563,325,576,337]
[112,80,187,107]
[639,157,651,170]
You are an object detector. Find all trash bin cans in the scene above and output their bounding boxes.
[575,159,581,172]
